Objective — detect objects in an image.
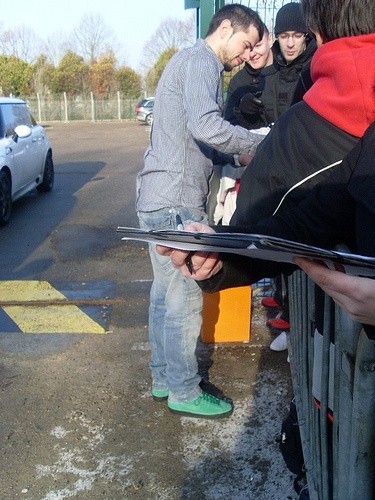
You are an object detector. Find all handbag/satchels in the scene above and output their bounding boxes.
[280,390,334,499]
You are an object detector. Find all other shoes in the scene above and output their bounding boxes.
[261,297,280,309]
[266,318,289,331]
[270,331,288,351]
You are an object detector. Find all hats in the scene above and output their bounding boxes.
[276,2,312,38]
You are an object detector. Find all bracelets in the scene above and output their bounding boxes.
[234,155,242,167]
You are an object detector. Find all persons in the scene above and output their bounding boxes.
[136,4,268,419]
[157,121,375,500]
[230,0,375,226]
[223,2,318,363]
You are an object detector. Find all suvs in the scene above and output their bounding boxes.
[137,97,155,125]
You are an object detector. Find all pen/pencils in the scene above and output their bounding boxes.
[175,213,194,276]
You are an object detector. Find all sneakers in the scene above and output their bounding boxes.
[167,389,233,418]
[150,385,167,401]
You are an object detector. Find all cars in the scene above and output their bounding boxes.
[0,94,53,221]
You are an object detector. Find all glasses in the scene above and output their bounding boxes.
[275,33,309,42]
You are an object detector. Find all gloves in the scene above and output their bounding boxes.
[238,90,264,115]
